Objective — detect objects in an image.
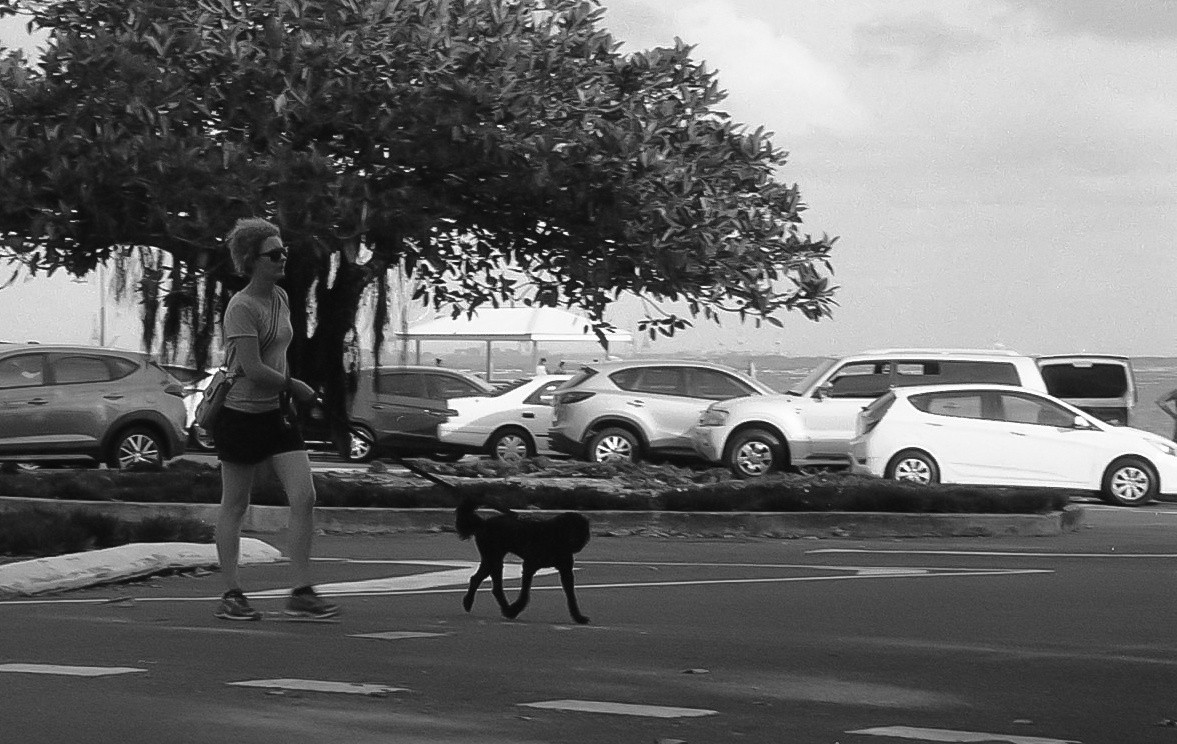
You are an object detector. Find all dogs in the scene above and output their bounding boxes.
[456,493,591,625]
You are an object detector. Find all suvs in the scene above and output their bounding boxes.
[545,359,785,464]
[695,347,1048,481]
[1034,352,1137,427]
[0,341,188,470]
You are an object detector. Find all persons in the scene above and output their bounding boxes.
[214,217,339,621]
[536,359,548,377]
[433,358,442,367]
[554,362,567,375]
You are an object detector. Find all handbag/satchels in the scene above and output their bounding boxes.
[195,371,232,430]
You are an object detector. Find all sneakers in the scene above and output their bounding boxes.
[213,594,261,620]
[283,594,340,618]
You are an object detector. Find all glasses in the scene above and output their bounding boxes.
[257,245,288,263]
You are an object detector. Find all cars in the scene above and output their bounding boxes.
[435,373,574,462]
[846,383,1177,507]
[299,364,502,462]
[483,379,519,392]
[158,363,226,451]
